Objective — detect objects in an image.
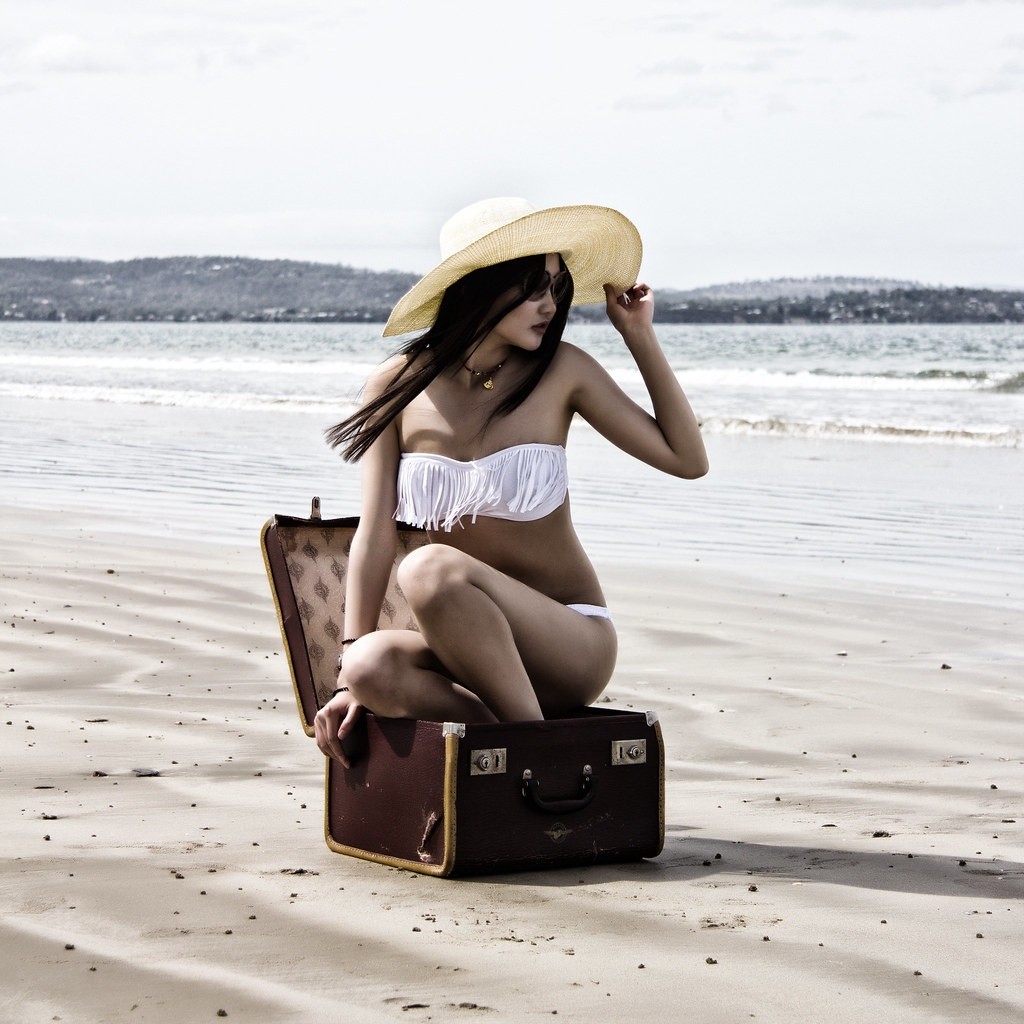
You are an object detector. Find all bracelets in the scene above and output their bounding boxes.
[333,686,349,697]
[342,638,359,645]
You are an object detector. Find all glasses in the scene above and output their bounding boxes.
[521,269,571,304]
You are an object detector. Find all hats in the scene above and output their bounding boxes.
[383,197,642,337]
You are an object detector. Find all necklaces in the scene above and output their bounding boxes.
[463,353,508,392]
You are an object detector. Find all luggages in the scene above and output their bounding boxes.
[259,497,665,879]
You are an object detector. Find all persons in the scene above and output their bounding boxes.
[314,194,711,770]
